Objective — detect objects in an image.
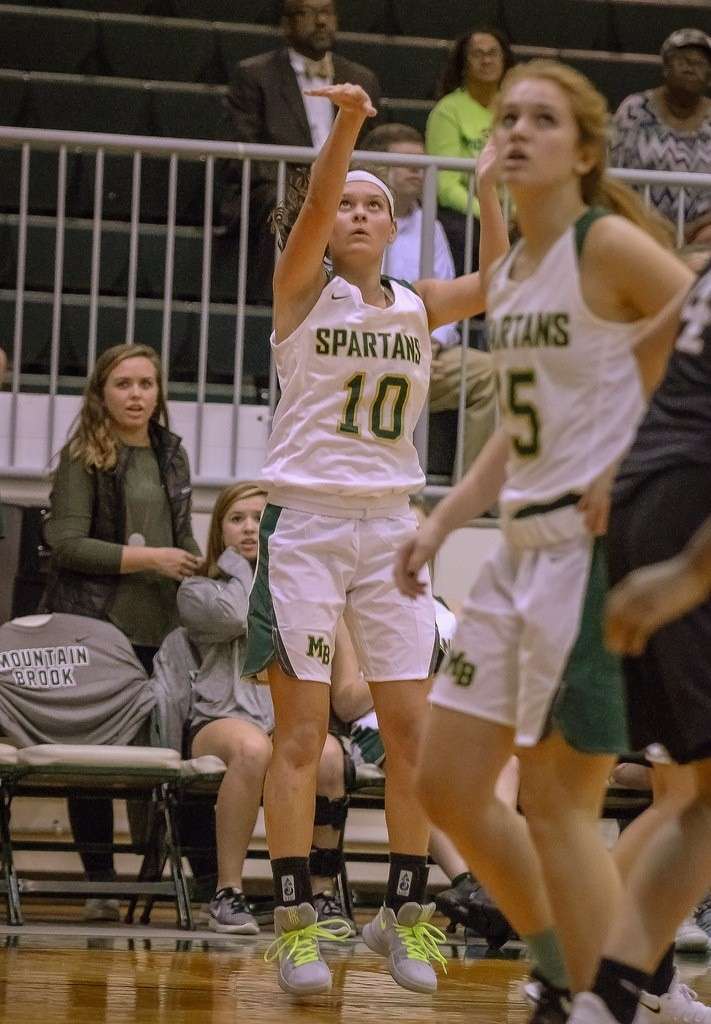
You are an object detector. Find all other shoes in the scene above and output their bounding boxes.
[692,896,711,934]
[84,894,121,921]
[520,975,575,1024]
[467,886,516,930]
[435,873,484,930]
[674,913,710,953]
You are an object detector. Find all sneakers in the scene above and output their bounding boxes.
[208,887,260,934]
[362,900,448,994]
[313,891,357,936]
[636,964,711,1024]
[263,902,351,994]
[565,992,667,1024]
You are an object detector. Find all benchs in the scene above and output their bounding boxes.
[0,0,711,405]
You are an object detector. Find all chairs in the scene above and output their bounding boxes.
[0,612,652,936]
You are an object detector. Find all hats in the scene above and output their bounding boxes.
[660,28,711,54]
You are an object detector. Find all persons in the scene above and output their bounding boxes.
[225,0,711,520]
[40,343,221,925]
[391,58,703,1024]
[238,83,508,1000]
[174,479,711,953]
[565,260,710,1024]
